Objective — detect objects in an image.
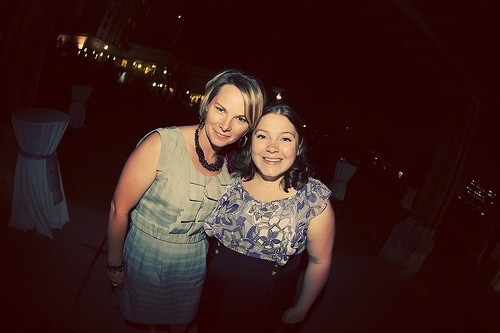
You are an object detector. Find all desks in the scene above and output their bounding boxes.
[7,105,72,240]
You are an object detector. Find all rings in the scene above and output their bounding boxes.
[112,282,118,287]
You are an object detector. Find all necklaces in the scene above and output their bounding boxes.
[195,121,224,171]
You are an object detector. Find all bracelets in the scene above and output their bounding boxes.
[105,260,125,273]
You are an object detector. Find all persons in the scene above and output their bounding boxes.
[105,71,263,333]
[198,105,336,333]
